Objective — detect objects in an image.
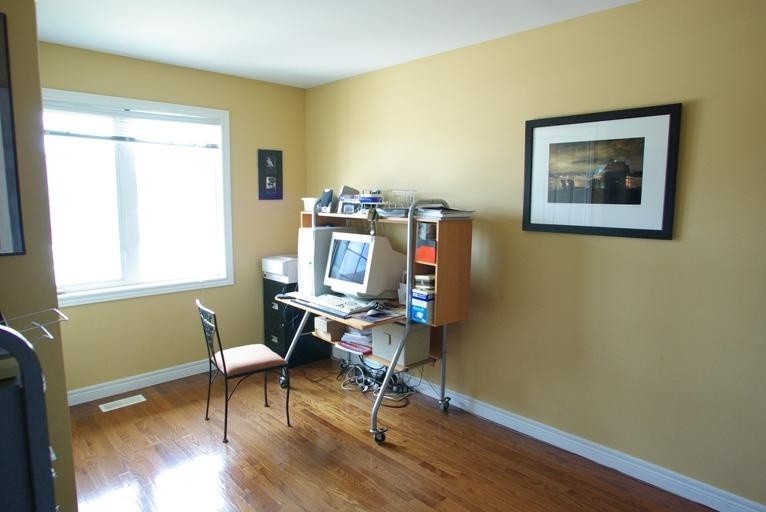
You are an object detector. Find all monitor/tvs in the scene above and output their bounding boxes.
[323,231,406,303]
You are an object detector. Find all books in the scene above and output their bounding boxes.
[337,328,374,349]
[419,206,476,219]
[412,276,434,301]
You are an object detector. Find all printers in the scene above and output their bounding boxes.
[262,255,297,284]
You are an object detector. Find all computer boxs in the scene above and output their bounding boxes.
[297,226,355,297]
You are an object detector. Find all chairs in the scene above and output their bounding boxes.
[196,298,291,443]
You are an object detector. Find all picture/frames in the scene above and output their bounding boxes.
[258,149,283,200]
[521,101,683,239]
[1,12,26,258]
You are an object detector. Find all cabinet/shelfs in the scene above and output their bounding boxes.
[298,195,477,327]
[262,279,331,367]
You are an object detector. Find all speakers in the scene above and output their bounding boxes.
[321,189,338,213]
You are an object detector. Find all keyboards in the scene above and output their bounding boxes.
[310,293,368,314]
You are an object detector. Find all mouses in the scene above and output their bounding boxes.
[367,309,387,316]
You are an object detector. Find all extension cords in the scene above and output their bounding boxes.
[393,383,408,393]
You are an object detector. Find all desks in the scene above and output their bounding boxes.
[274,291,452,445]
